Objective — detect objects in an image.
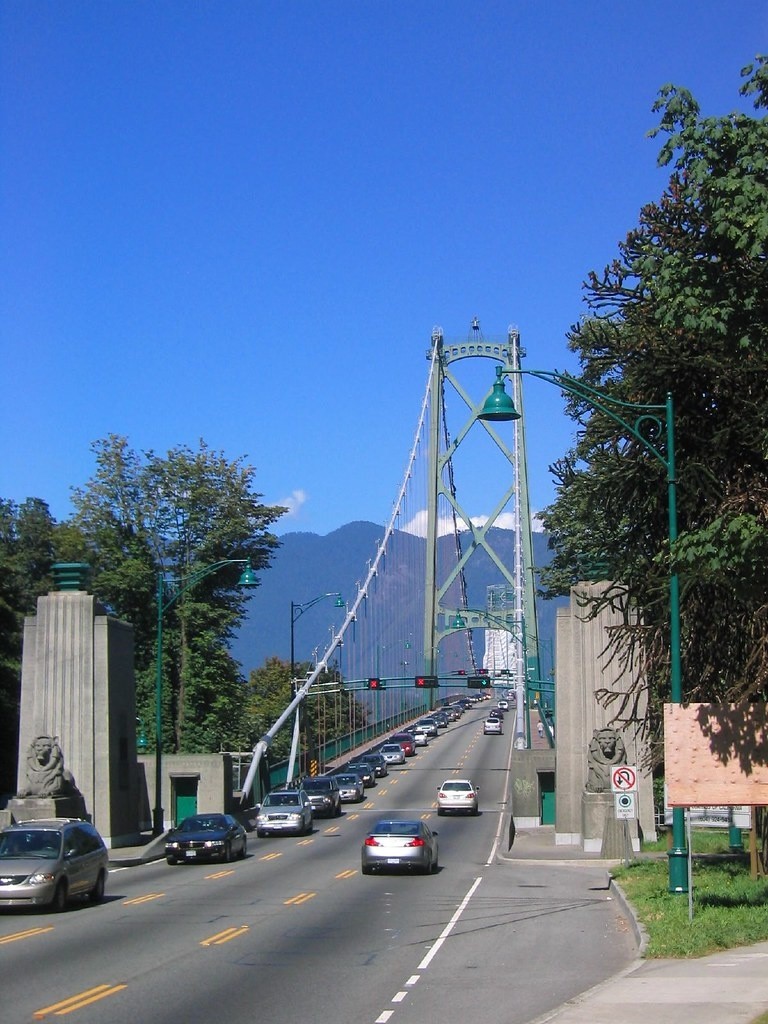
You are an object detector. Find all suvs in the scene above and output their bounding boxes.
[415,718,438,737]
[387,733,415,757]
[255,789,312,839]
[301,776,341,819]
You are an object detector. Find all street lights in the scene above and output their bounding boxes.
[375,638,414,734]
[472,365,692,894]
[510,631,556,738]
[152,555,262,838]
[450,607,531,750]
[290,591,345,740]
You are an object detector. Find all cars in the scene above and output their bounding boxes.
[164,812,248,866]
[360,754,387,778]
[438,779,480,816]
[429,691,515,728]
[379,744,406,764]
[0,818,108,914]
[348,763,375,788]
[483,717,503,735]
[411,730,428,746]
[360,818,438,875]
[332,774,365,803]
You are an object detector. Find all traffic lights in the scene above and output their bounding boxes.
[467,676,490,689]
[479,669,488,674]
[368,678,380,690]
[459,670,465,675]
[415,675,438,688]
[501,670,509,674]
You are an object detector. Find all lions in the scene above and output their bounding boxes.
[16,734,81,799]
[584,727,628,793]
